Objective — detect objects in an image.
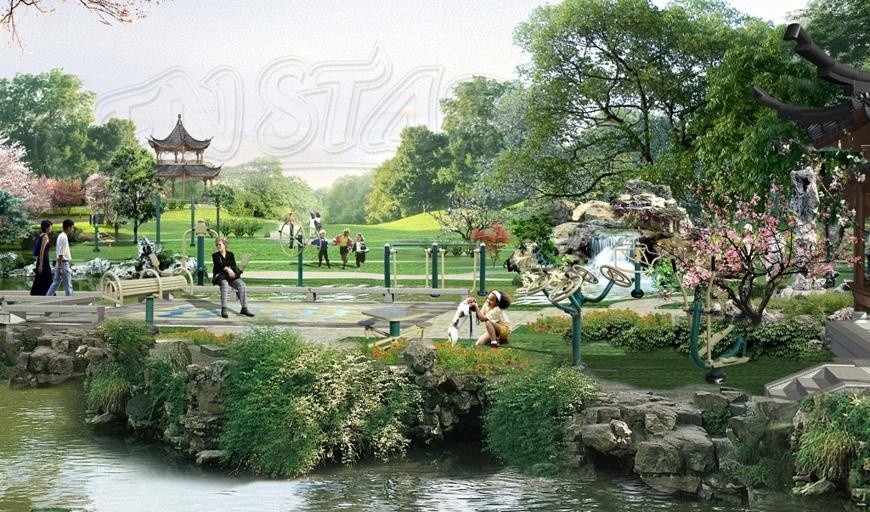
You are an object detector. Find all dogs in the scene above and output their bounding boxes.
[448,296,478,347]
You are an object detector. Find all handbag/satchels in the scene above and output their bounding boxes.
[32,234,50,256]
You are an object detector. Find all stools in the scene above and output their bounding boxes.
[359,318,390,340]
[401,321,433,342]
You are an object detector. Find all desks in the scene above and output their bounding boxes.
[358,307,433,351]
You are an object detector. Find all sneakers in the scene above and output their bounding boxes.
[221,308,228,318]
[240,309,254,317]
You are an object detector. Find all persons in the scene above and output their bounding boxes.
[284,208,369,269]
[469,290,513,349]
[210,234,254,321]
[30,218,52,296]
[45,219,74,298]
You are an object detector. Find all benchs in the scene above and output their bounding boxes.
[187,285,470,302]
